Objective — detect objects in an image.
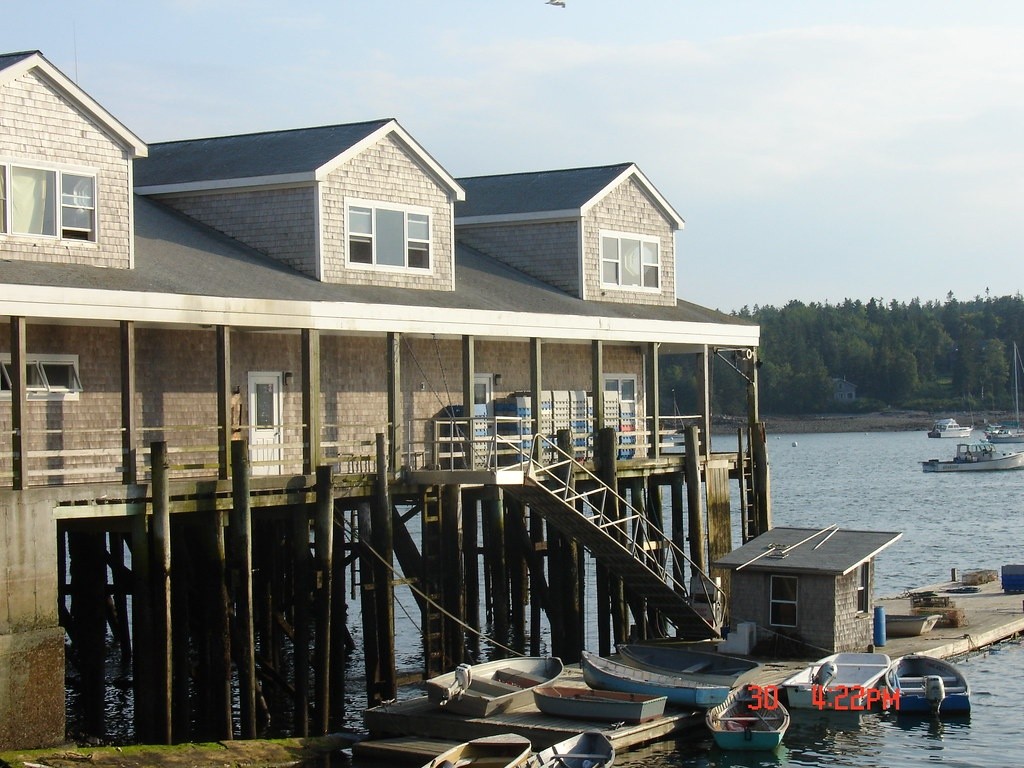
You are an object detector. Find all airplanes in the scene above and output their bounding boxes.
[544,0,568,9]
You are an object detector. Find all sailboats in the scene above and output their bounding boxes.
[985,341,1024,443]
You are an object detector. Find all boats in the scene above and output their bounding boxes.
[917,443,1024,472]
[615,643,761,687]
[885,613,944,637]
[426,656,564,717]
[928,418,973,437]
[982,424,1012,438]
[781,653,891,710]
[706,680,790,752]
[514,727,616,768]
[529,685,670,724]
[418,733,532,768]
[884,653,971,715]
[580,650,731,710]
